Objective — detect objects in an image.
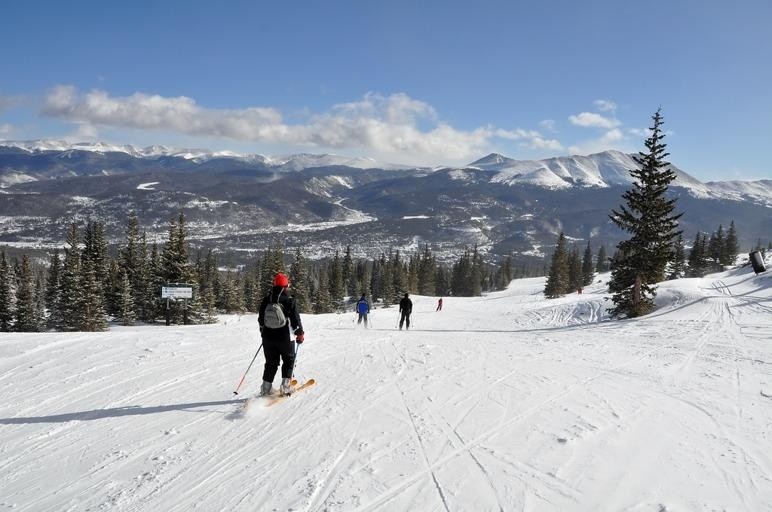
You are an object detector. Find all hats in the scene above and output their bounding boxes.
[274,273,288,287]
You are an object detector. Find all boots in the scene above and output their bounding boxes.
[281,378,294,393]
[262,381,275,395]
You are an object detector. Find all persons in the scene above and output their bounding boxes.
[436,298,442,310]
[257,273,304,395]
[356,293,370,326]
[399,292,412,329]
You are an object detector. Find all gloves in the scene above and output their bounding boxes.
[296,334,304,343]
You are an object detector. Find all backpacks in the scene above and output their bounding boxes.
[264,288,286,329]
